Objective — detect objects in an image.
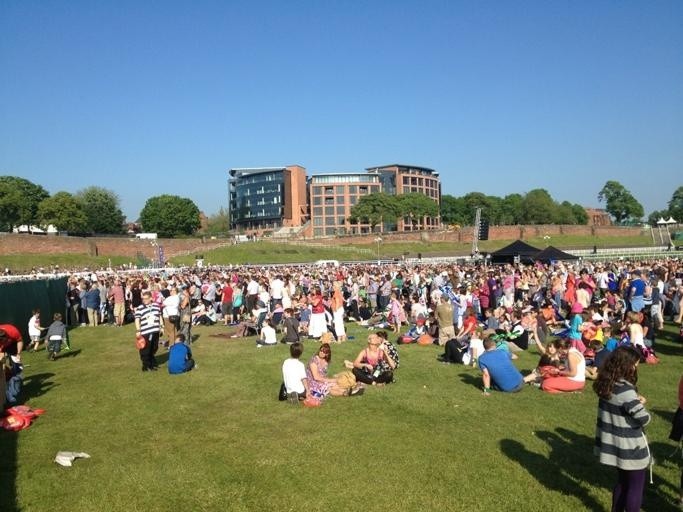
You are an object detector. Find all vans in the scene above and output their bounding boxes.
[313,260,339,269]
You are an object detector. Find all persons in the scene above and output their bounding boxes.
[1,260,682,406]
[666,241,672,250]
[591,345,652,512]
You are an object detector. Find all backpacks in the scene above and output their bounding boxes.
[6,376,26,404]
[444,338,463,362]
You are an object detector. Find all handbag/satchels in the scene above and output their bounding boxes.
[183,314,190,322]
[169,316,179,323]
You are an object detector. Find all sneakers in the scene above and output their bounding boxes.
[286,391,299,404]
[343,386,364,397]
[230,335,239,338]
[141,367,157,372]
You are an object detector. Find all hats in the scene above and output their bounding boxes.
[571,303,583,313]
[257,301,264,307]
[626,270,641,276]
[441,293,449,301]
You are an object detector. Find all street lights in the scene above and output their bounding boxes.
[374,237,382,265]
[543,235,550,248]
[150,239,158,268]
